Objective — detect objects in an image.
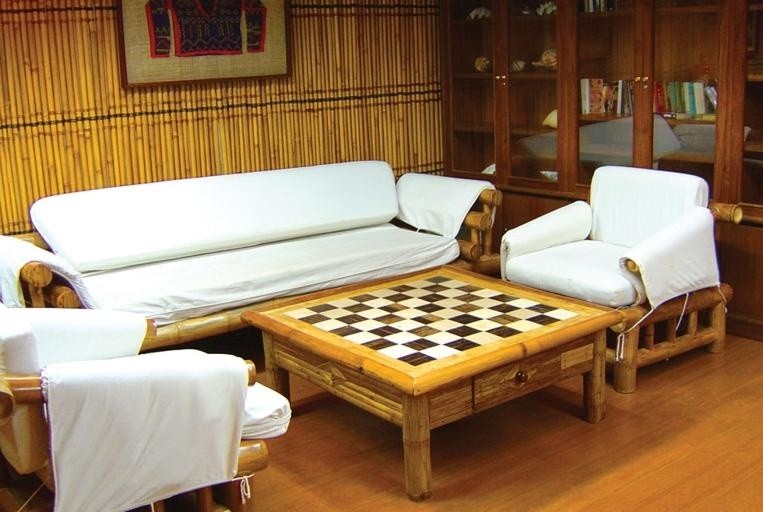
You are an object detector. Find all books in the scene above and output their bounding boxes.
[579,77,719,117]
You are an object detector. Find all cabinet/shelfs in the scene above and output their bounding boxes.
[568,0,730,336]
[436,1,568,272]
[730,0,763,345]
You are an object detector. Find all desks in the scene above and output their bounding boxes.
[239,261,629,504]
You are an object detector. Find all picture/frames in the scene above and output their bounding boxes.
[113,0,297,90]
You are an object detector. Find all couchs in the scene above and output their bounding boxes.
[498,164,746,396]
[0,306,293,511]
[0,159,505,361]
[511,109,753,194]
